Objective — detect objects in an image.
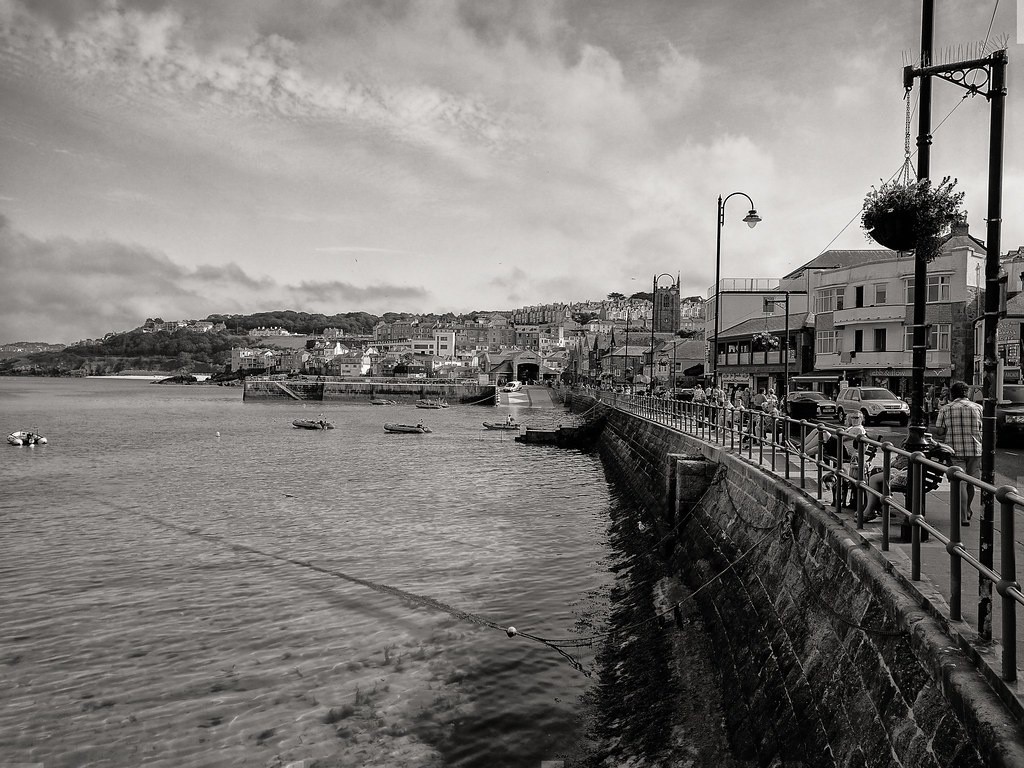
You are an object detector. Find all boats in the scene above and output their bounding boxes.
[291,418,335,430]
[384,423,434,434]
[7,431,49,446]
[371,398,396,405]
[483,421,521,430]
[415,399,451,409]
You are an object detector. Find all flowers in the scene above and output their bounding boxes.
[858,176,968,264]
[752,331,780,349]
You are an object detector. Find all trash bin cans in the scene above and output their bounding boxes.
[675,393,694,414]
[787,397,819,437]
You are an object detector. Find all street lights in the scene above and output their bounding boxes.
[712,191,763,394]
[649,270,678,397]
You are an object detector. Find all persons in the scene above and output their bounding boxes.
[853,423,911,523]
[538,375,780,450]
[924,391,948,424]
[785,410,867,461]
[936,381,980,527]
[506,414,512,425]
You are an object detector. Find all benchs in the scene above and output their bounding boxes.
[766,416,784,445]
[823,434,883,468]
[735,413,749,434]
[890,454,947,518]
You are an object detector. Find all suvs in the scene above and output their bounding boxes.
[835,386,911,428]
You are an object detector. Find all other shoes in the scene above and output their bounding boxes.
[961,520,970,526]
[784,438,806,455]
[967,511,972,519]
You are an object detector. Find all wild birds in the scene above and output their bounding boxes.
[355,258,357,262]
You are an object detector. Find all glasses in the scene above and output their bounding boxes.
[850,417,859,420]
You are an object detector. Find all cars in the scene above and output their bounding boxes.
[503,380,523,392]
[780,391,837,421]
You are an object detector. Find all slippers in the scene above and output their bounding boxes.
[854,512,877,523]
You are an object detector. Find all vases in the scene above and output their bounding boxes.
[868,226,917,252]
